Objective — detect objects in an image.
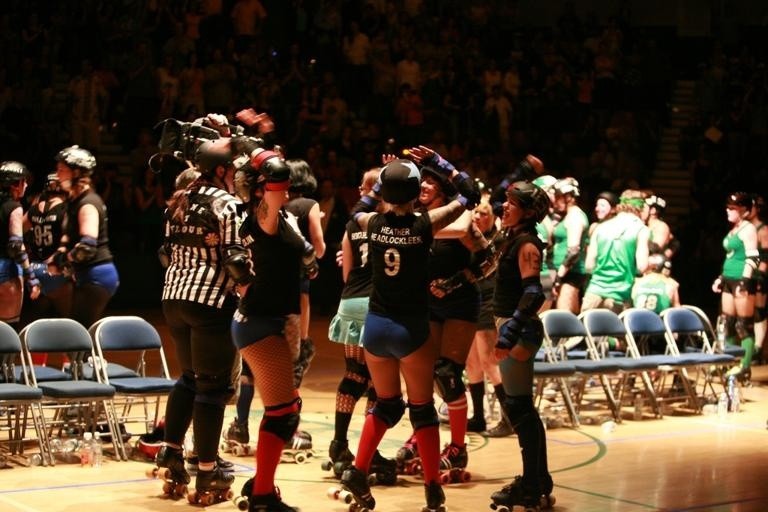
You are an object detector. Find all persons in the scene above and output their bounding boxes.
[1,144,120,382]
[339,143,480,509]
[395,162,498,471]
[135,110,327,511]
[712,189,767,381]
[326,165,396,470]
[532,173,680,352]
[0,1,768,308]
[464,195,516,438]
[428,177,554,506]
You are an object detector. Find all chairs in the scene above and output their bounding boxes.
[0,316,189,468]
[489,307,735,429]
[681,304,746,403]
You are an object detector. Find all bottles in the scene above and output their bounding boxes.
[146,411,157,433]
[88,355,108,369]
[27,431,104,470]
[633,393,643,422]
[702,375,741,418]
[578,413,618,435]
[30,262,50,276]
[538,406,549,431]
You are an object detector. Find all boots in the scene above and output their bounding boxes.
[467,381,515,437]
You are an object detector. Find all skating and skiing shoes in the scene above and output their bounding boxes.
[144,418,312,512]
[321,433,470,512]
[490,475,556,512]
[708,363,752,387]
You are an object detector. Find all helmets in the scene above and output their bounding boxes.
[726,191,764,209]
[378,159,581,223]
[0,144,98,182]
[594,191,667,210]
[650,253,672,272]
[195,137,234,178]
